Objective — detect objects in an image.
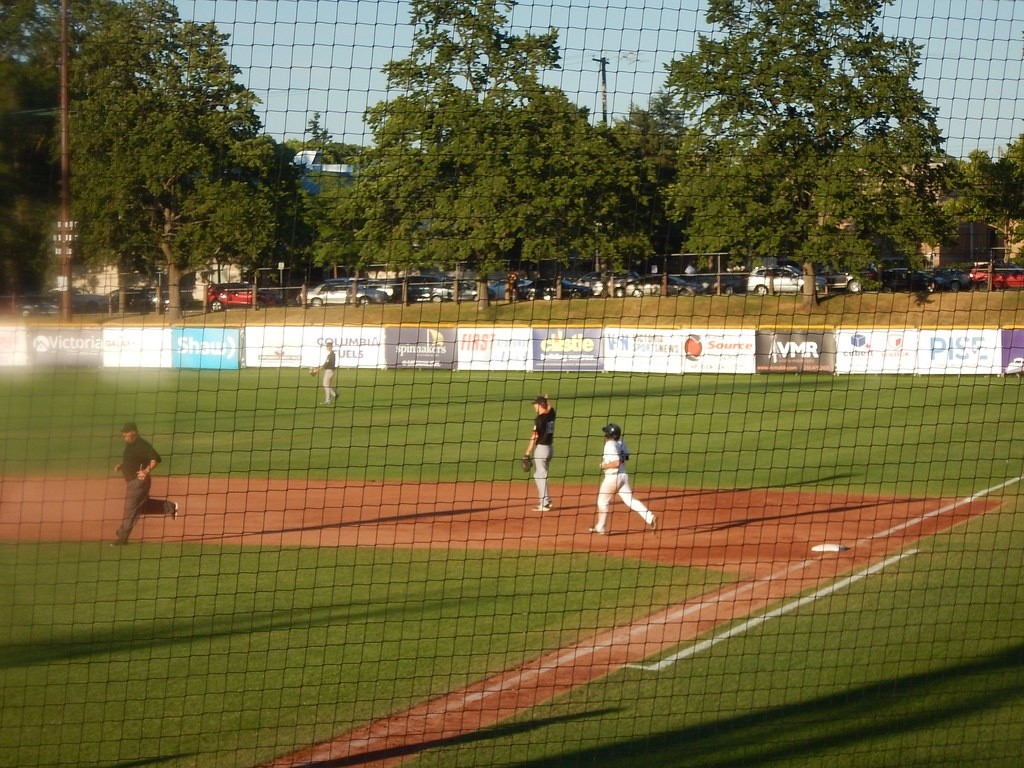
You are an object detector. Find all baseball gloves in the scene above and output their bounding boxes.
[521,454,533,473]
[309,367,317,377]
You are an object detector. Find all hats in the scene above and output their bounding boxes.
[326,343,333,347]
[120,423,137,432]
[531,396,547,405]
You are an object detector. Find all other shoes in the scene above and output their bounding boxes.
[588,528,605,535]
[172,502,178,520]
[332,395,338,401]
[532,506,550,511]
[319,401,330,404]
[651,516,657,530]
[111,540,128,546]
[537,503,553,508]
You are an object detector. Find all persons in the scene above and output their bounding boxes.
[520,393,556,512]
[587,423,658,536]
[310,343,340,405]
[110,422,178,546]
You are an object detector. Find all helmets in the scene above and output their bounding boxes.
[602,423,621,440]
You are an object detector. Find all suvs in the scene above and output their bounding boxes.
[747,266,825,295]
[969,262,1024,291]
[207,281,276,312]
[821,265,878,293]
[573,271,639,297]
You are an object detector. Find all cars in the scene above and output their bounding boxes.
[17,289,170,317]
[298,278,437,308]
[490,278,533,300]
[880,268,968,294]
[517,278,592,301]
[687,270,743,294]
[624,275,701,297]
[432,282,494,302]
